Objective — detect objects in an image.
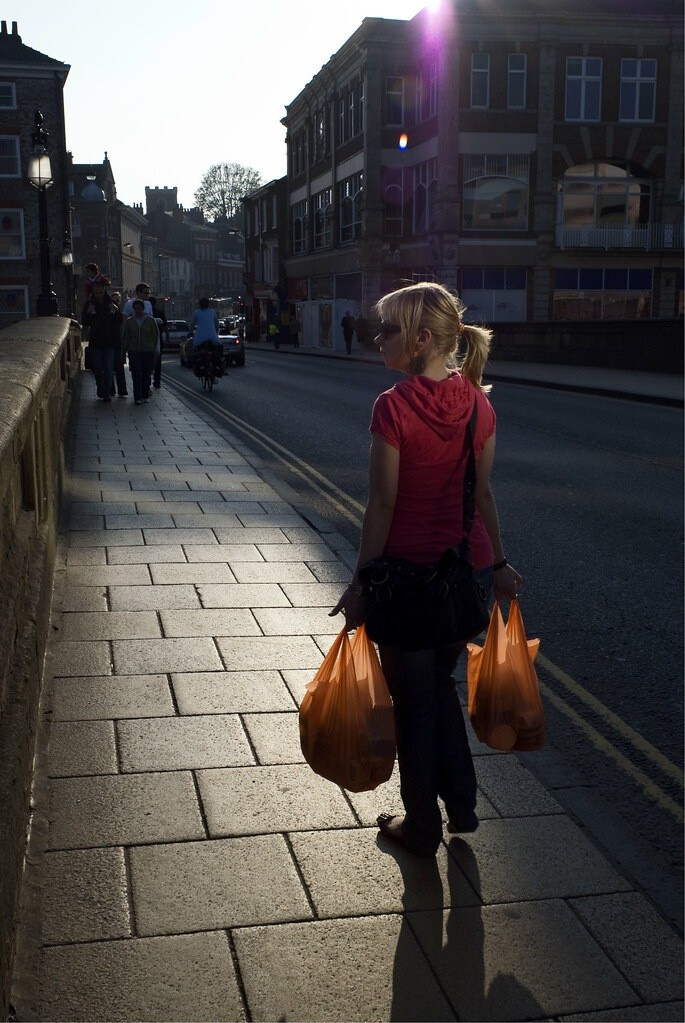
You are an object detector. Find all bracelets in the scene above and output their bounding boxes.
[346,583,370,596]
[493,557,507,571]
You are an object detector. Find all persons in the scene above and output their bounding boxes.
[272,315,281,348]
[341,310,355,355]
[287,314,301,348]
[124,299,159,404]
[186,297,219,385]
[79,262,168,401]
[328,282,527,848]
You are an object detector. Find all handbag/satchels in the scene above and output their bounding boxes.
[466,595,546,752]
[357,548,490,650]
[85,347,102,369]
[299,621,395,793]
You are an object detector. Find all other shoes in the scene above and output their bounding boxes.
[144,398,150,403]
[376,812,437,859]
[136,400,144,404]
[447,814,479,832]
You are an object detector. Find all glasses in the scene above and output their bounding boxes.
[377,321,434,342]
[143,291,151,295]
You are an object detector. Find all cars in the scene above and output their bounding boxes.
[178,313,247,369]
[161,319,190,348]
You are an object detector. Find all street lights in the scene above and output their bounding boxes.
[24,109,60,317]
[214,228,240,318]
[61,229,75,320]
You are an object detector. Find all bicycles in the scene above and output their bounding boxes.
[192,345,219,391]
